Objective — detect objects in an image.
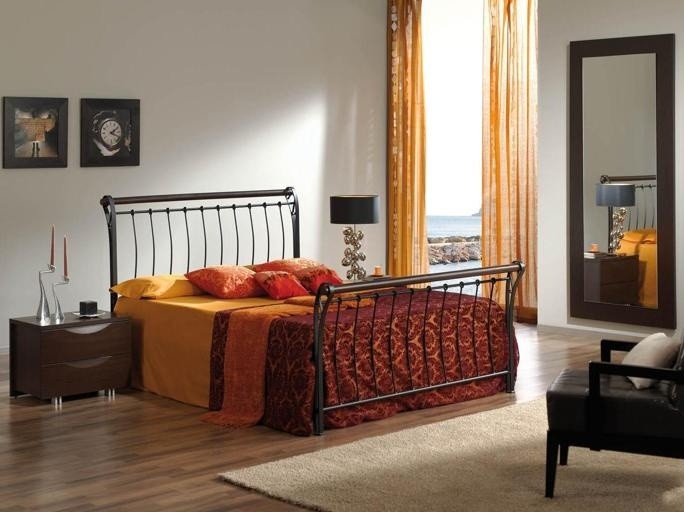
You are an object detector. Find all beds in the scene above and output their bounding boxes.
[600,174,657,309]
[101,187,524,435]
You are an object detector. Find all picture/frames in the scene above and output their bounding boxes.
[80,98,140,168]
[3,96,68,169]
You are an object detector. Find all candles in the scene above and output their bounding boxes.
[50,224,55,267]
[64,236,68,277]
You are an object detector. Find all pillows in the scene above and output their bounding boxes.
[108,258,343,300]
[620,333,681,389]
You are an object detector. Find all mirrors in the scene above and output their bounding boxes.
[569,34,677,328]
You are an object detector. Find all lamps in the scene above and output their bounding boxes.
[596,184,635,253]
[329,195,380,280]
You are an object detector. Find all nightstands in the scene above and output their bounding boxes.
[583,255,639,306]
[9,310,134,403]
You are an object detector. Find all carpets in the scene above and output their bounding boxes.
[217,395,684,512]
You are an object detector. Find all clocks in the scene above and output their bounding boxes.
[98,117,124,151]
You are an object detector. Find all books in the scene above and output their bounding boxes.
[363,275,392,281]
[581,251,608,258]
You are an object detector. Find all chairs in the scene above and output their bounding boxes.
[544,334,684,499]
